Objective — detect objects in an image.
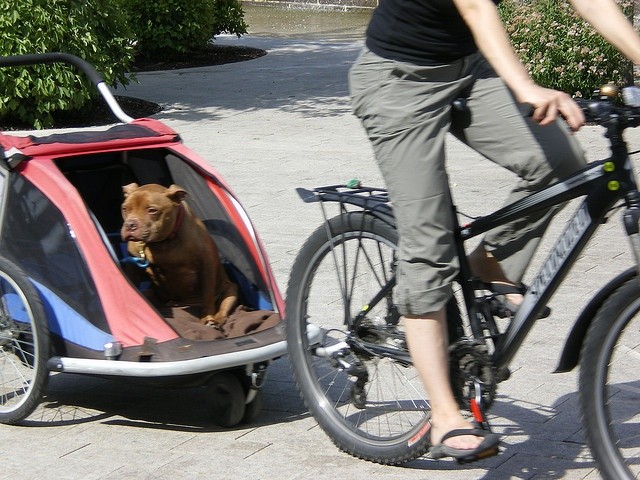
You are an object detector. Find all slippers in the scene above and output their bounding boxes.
[473,282,551,319]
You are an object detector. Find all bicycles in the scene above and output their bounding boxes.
[283,84,640,479]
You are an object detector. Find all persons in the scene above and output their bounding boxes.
[349,0,640,458]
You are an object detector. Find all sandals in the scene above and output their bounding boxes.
[431,428,499,459]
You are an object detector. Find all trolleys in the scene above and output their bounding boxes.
[1,54,289,427]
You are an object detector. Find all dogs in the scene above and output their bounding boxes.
[120,182,241,329]
